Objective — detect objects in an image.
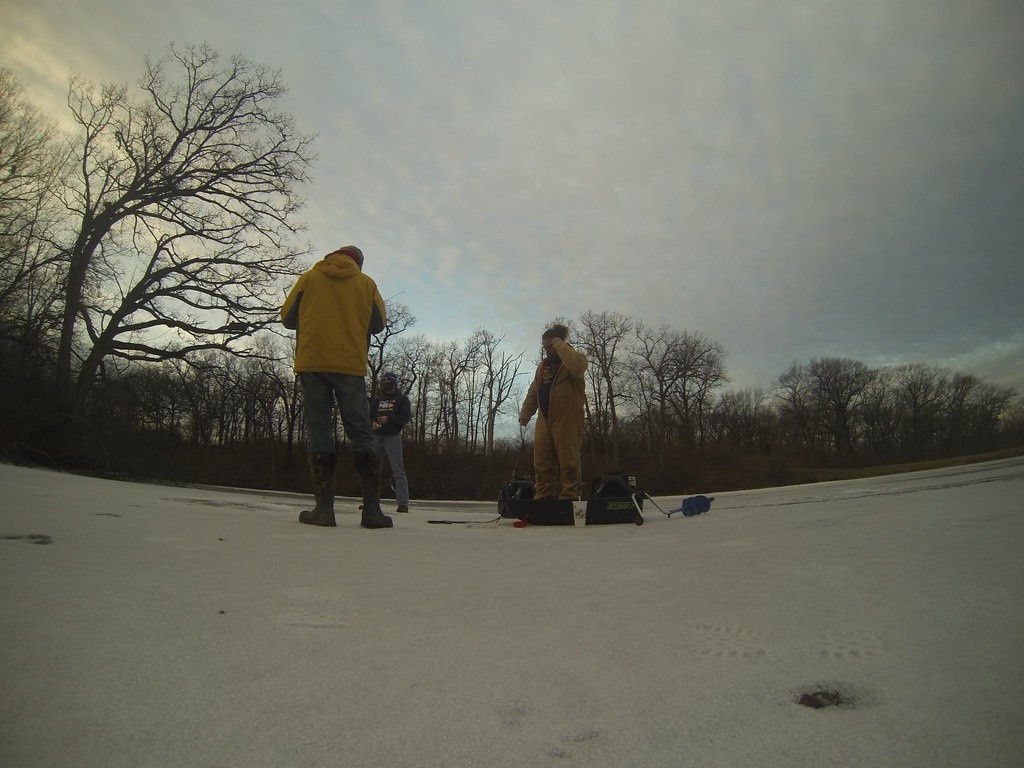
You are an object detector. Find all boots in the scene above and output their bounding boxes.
[299,498,336,527]
[359,504,393,527]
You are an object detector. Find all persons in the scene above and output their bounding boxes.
[518,324,588,503]
[359,372,408,513]
[280,245,392,529]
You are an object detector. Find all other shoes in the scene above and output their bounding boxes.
[397,504,408,513]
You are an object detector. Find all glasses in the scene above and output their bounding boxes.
[383,377,391,383]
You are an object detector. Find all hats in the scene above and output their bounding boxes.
[342,244,364,262]
[383,372,397,385]
